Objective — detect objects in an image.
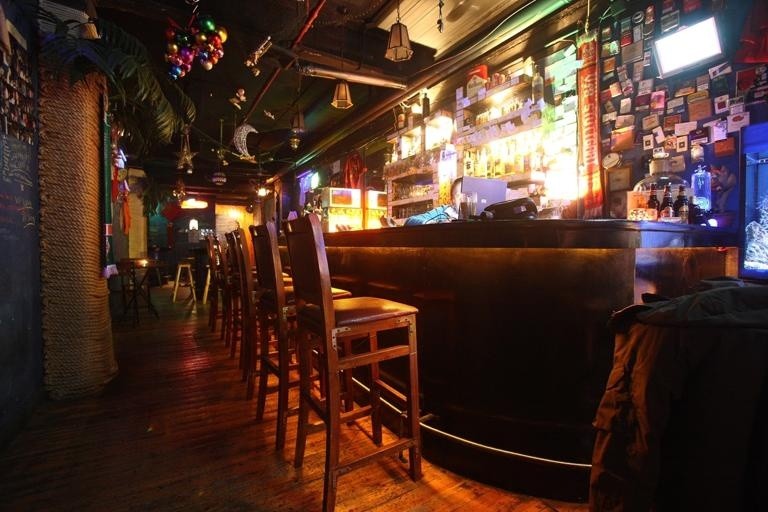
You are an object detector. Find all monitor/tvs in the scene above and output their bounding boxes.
[650,13,727,81]
[310,171,319,190]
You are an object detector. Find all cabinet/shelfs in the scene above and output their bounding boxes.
[384,109,456,220]
[454,74,547,212]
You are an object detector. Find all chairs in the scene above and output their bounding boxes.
[106,223,293,394]
[280,212,421,511]
[246,222,354,468]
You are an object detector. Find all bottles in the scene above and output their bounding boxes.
[462,145,538,179]
[531,71,544,102]
[397,107,406,129]
[646,182,689,224]
[422,93,430,120]
[681,164,714,214]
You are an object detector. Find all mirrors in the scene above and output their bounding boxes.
[362,135,391,229]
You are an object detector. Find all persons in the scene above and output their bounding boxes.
[405,177,462,225]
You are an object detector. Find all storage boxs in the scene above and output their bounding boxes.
[320,205,363,233]
[364,190,388,211]
[363,208,387,230]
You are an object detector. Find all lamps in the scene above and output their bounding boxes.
[328,6,354,111]
[384,1,414,63]
[291,62,306,134]
[211,118,229,186]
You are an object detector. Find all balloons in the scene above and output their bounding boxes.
[163,14,228,83]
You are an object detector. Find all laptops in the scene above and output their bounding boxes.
[436,176,508,222]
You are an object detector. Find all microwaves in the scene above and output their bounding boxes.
[364,190,387,209]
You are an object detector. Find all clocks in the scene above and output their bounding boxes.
[602,164,634,220]
